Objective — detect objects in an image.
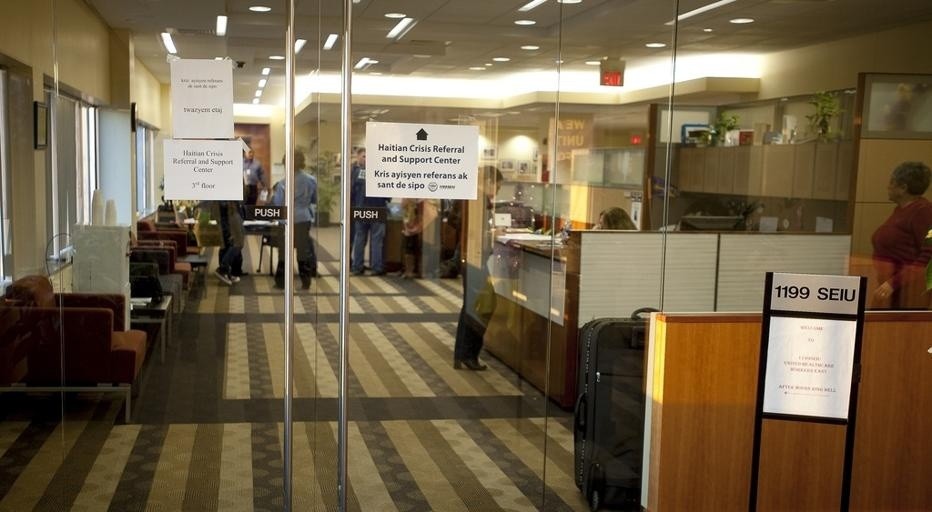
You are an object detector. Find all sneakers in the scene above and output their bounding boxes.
[215,267,232,286]
[231,275,241,284]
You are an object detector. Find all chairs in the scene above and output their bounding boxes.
[1,276,148,425]
[132,219,205,290]
[130,262,184,316]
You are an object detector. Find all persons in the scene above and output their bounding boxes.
[243,148,269,205]
[350,148,461,279]
[155,196,248,286]
[272,150,317,289]
[871,161,932,309]
[454,166,503,371]
[592,207,638,230]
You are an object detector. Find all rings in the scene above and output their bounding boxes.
[881,293,885,296]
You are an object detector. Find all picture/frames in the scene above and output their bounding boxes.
[479,147,499,161]
[517,160,534,177]
[33,101,50,149]
[499,158,516,173]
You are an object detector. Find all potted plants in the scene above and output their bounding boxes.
[804,89,842,136]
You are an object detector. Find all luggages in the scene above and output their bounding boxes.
[574,306,658,512]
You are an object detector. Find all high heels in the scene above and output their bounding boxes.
[455,358,487,370]
